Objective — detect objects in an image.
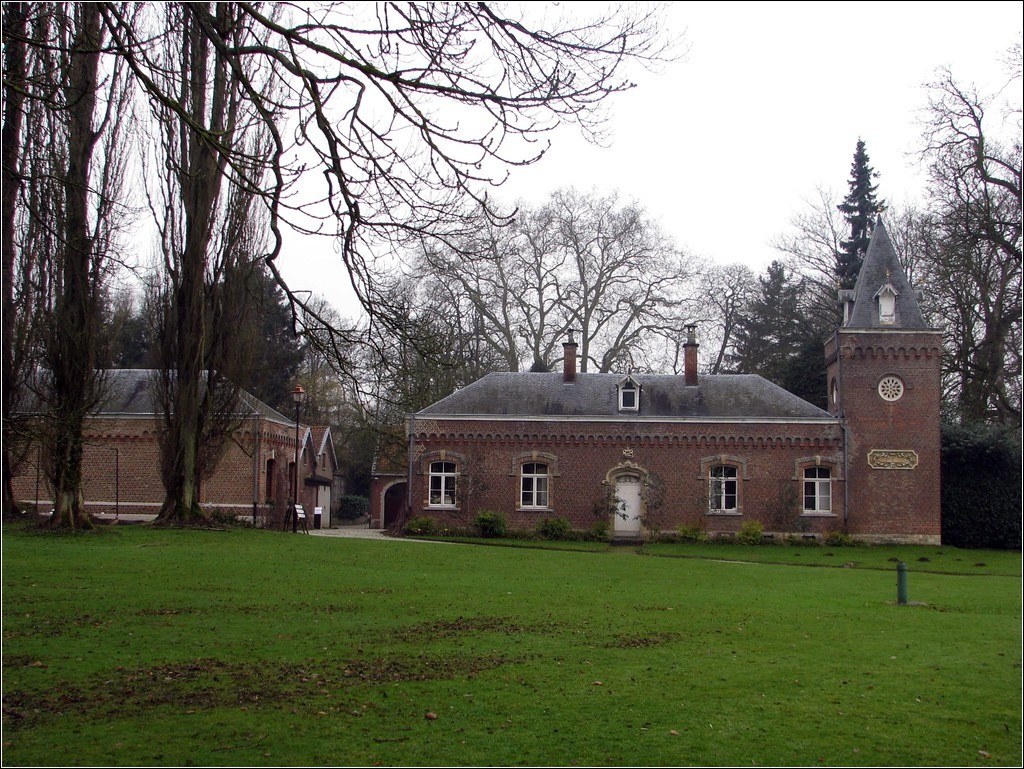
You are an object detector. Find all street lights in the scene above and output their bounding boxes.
[110,447,120,515]
[291,383,307,533]
